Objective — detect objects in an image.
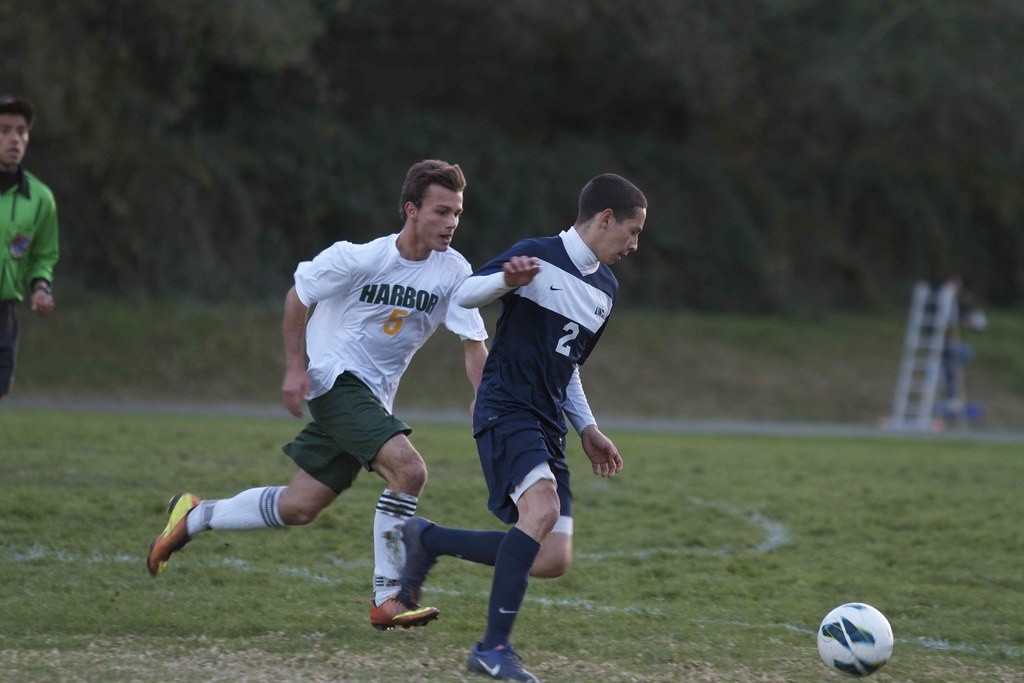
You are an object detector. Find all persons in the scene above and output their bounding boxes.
[0,97,59,398]
[144,158,490,633]
[400,173,648,683]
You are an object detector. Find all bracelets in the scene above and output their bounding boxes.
[33,285,51,294]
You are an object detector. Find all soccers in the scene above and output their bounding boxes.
[817,602,894,678]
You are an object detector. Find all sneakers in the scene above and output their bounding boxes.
[369,592,441,630]
[398,517,439,606]
[146,492,202,576]
[466,640,540,683]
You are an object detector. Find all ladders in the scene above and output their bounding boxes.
[891,277,986,428]
